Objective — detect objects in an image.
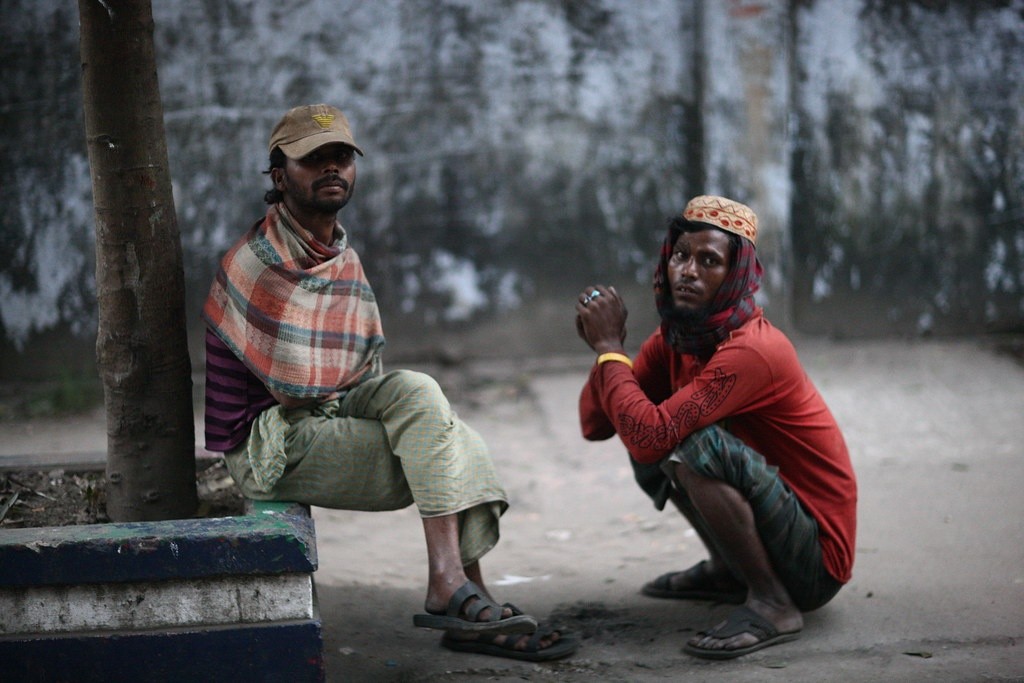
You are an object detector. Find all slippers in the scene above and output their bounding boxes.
[441,626,581,659]
[413,579,538,634]
[645,559,749,604]
[686,600,804,658]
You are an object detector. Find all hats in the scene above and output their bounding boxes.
[683,196,759,248]
[268,105,364,173]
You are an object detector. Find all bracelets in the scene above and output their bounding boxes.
[598,353,633,369]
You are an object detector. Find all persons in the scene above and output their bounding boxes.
[206,103,576,658]
[576,196,853,654]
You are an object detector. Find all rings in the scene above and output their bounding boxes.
[584,297,592,304]
[591,290,600,297]
